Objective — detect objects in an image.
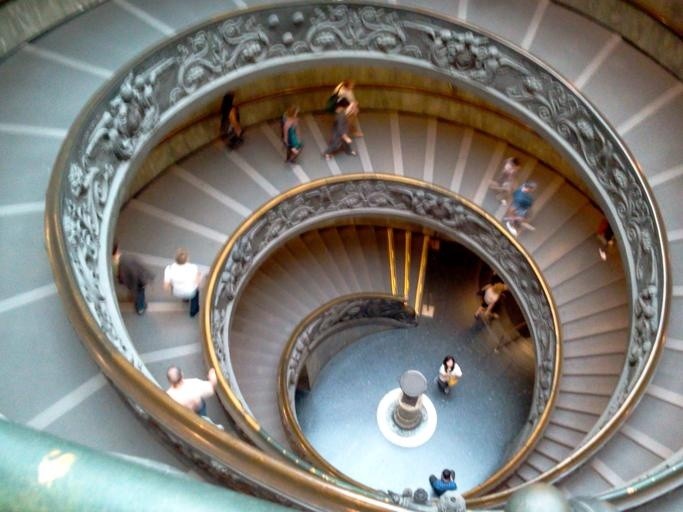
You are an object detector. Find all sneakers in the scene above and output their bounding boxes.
[343,148,357,157]
[598,247,606,261]
[137,303,147,315]
[505,223,517,238]
[320,152,332,161]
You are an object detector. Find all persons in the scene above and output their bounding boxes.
[487,156,524,206]
[281,78,364,164]
[112,238,156,315]
[434,355,462,394]
[166,367,217,416]
[219,91,245,151]
[163,249,202,317]
[597,216,614,261]
[505,180,538,237]
[474,283,504,319]
[403,469,457,505]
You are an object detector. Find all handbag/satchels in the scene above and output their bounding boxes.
[447,376,458,388]
[324,94,339,115]
